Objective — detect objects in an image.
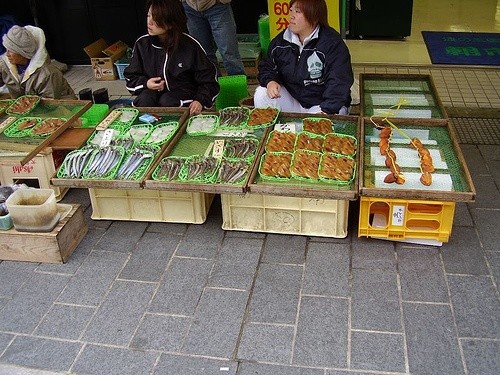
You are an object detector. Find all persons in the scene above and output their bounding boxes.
[123,0,221,116]
[179,0,246,76]
[0,25,79,100]
[255,0,354,116]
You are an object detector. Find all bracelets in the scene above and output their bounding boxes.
[320,111,327,115]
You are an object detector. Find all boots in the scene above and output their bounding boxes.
[79,87,112,109]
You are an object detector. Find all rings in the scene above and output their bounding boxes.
[158,90,160,91]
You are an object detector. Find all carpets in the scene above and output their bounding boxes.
[421,30,500,65]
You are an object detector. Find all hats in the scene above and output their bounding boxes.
[2,25,37,60]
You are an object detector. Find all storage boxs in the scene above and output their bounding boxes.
[0,73,476,264]
[84,39,132,80]
